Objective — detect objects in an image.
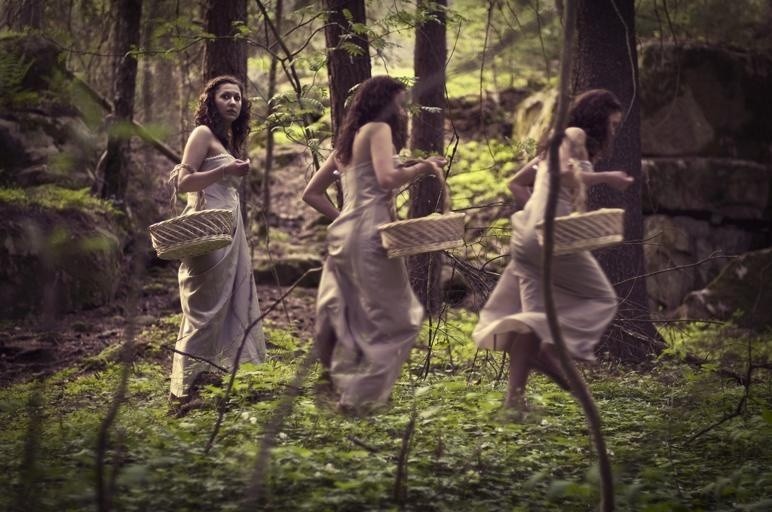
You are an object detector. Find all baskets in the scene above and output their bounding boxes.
[376,159,466,259]
[147,163,233,261]
[534,158,626,257]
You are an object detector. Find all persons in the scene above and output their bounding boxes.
[470,88,639,425]
[300,76,449,417]
[170,75,269,402]
